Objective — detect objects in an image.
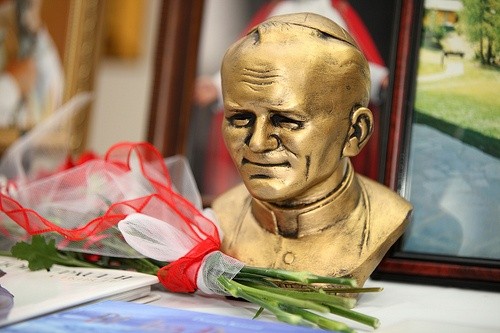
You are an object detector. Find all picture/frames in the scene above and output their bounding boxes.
[369,0,500,295]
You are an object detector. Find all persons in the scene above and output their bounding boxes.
[211,13,412,298]
[12,1,64,132]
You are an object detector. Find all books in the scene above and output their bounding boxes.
[0,253,332,333]
